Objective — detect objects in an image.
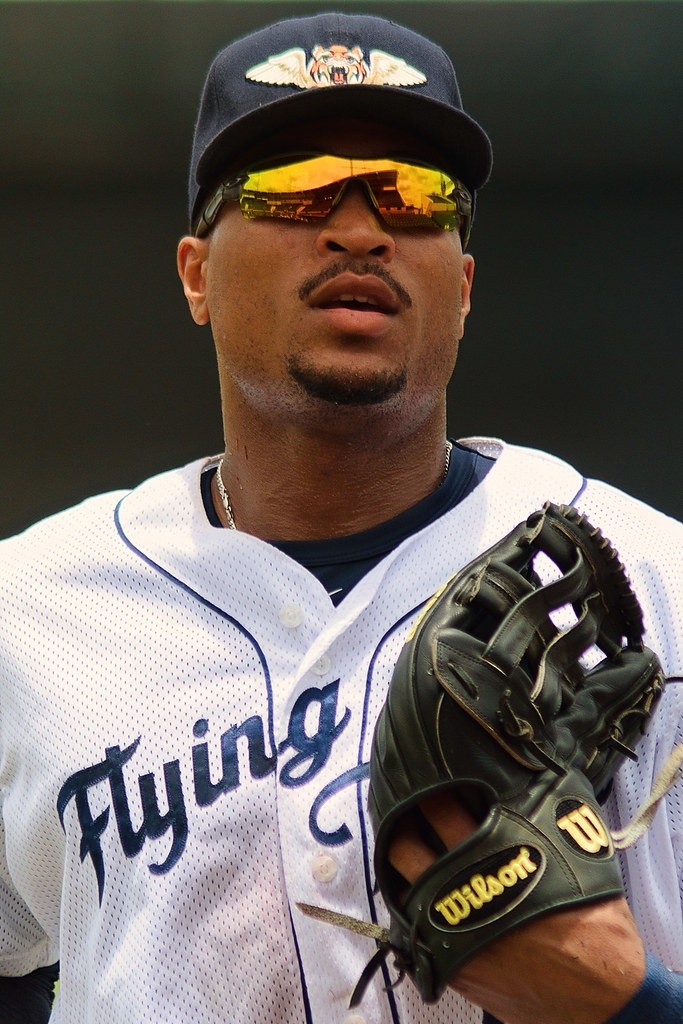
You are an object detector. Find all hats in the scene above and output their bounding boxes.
[186,10,494,253]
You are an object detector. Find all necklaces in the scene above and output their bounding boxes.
[216,441,453,530]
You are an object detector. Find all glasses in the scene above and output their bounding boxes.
[193,150,475,255]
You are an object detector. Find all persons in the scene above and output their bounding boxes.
[0,12,683,1023]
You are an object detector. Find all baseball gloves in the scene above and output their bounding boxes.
[292,498,683,1016]
